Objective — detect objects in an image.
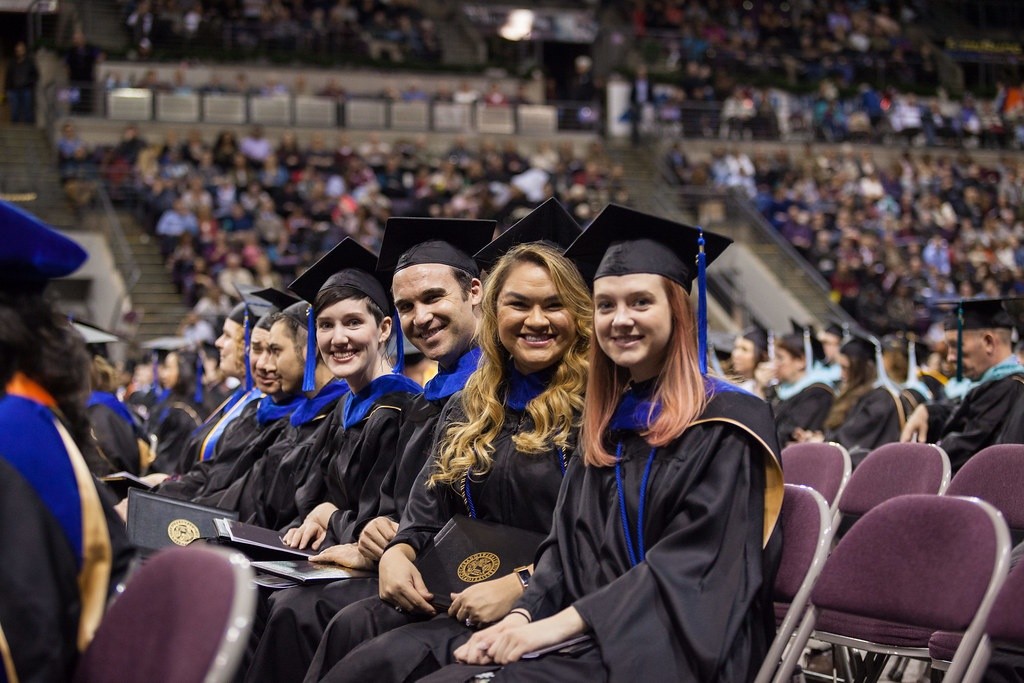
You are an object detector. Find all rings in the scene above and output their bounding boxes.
[465,618,474,626]
[395,605,402,612]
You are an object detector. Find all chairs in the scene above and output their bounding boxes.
[72,543,259,683]
[755,435,1024,683]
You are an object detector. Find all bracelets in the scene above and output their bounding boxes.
[509,607,532,623]
[514,566,532,589]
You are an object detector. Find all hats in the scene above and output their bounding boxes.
[69,316,118,345]
[932,298,1023,382]
[225,281,273,389]
[144,335,189,353]
[283,298,310,330]
[471,196,585,274]
[740,316,944,388]
[249,289,298,312]
[288,235,395,391]
[1,200,88,280]
[190,311,227,344]
[377,217,499,287]
[562,204,734,376]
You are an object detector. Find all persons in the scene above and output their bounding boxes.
[0,199,133,683]
[715,295,1024,483]
[110,194,792,683]
[71,314,233,502]
[52,123,629,344]
[4,43,42,126]
[609,0,1024,152]
[662,140,1024,340]
[55,29,107,116]
[120,0,601,126]
